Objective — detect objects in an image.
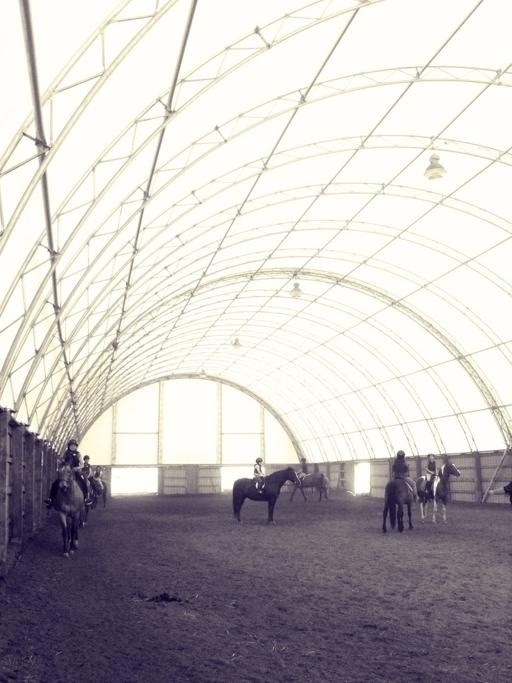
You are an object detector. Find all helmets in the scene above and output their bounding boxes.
[256,457,263,463]
[67,439,78,446]
[83,454,89,460]
[397,450,406,460]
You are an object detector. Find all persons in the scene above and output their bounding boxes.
[254,458,266,495]
[392,451,420,503]
[424,454,440,507]
[298,458,308,488]
[488,481,512,505]
[44,439,104,509]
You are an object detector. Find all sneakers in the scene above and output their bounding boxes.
[43,498,51,505]
[85,499,93,506]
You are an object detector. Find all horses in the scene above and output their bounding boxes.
[52,457,107,558]
[231,466,302,526]
[381,479,414,533]
[289,471,329,502]
[415,462,461,525]
